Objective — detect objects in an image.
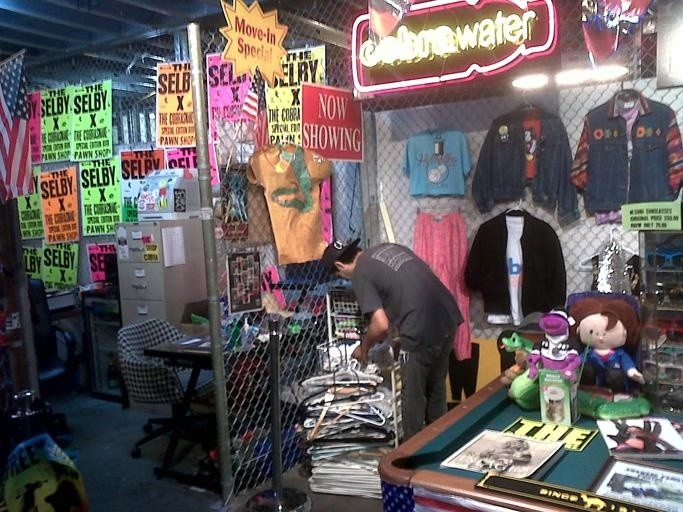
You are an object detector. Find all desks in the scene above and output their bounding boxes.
[143,327,316,494]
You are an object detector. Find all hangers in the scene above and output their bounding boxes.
[522,87,537,111]
[579,226,638,269]
[277,131,298,146]
[428,118,440,133]
[612,73,638,99]
[506,197,523,213]
[423,198,451,215]
[303,341,403,499]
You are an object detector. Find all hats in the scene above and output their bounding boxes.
[322,238,361,270]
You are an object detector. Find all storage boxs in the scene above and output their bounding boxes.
[621,202,681,230]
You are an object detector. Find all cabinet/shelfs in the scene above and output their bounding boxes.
[80,292,129,410]
[114,219,207,418]
[639,230,683,409]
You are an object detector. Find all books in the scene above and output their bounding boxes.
[595,416,683,461]
[441,429,566,483]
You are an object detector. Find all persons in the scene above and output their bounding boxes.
[322,235,464,440]
[569,292,646,390]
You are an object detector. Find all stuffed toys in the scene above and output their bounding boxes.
[527,305,582,384]
[499,332,651,419]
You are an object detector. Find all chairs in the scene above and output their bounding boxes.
[118,317,215,480]
